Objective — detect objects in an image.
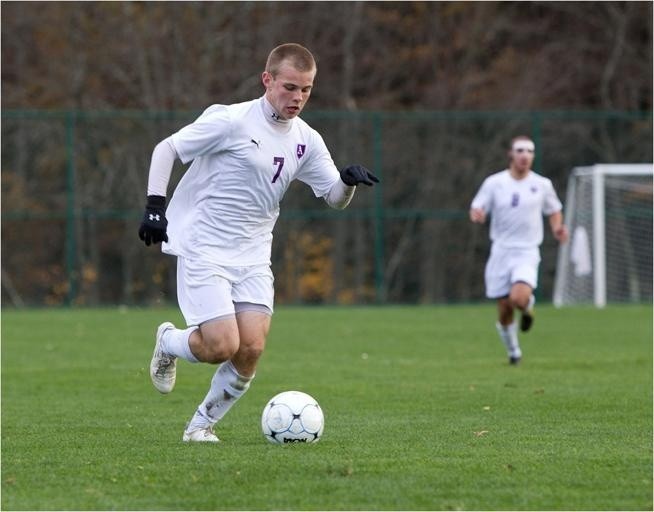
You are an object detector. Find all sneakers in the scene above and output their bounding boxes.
[150,322,176,394]
[520,313,533,331]
[182,425,220,442]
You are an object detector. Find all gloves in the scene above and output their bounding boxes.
[340,164,380,187]
[138,195,168,246]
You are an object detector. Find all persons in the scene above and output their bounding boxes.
[137,42,381,444]
[468,133,571,369]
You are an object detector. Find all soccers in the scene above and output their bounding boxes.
[261,390,324,444]
